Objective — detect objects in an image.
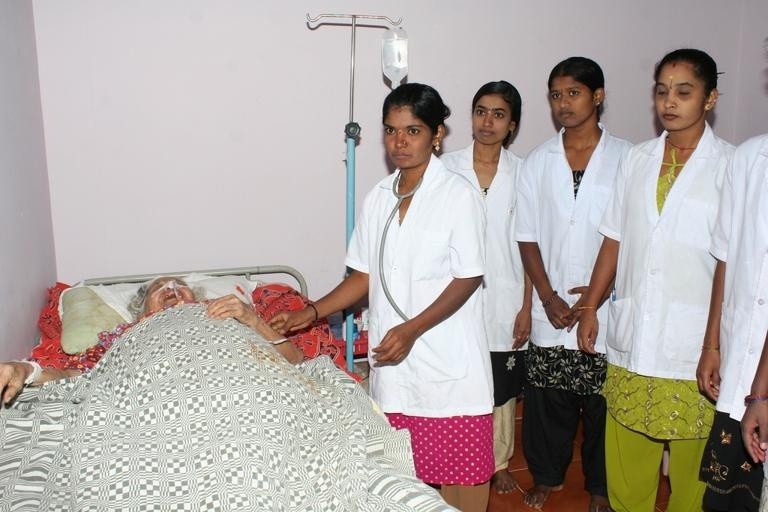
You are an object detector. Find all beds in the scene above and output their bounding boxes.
[1,265,460,512]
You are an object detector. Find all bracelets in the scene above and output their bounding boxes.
[307,303,321,324]
[743,394,768,404]
[580,305,598,312]
[542,290,559,308]
[701,345,720,354]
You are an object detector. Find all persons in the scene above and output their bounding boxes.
[697,67,768,511]
[513,56,641,512]
[0,276,305,411]
[739,329,768,512]
[434,80,533,495]
[266,84,495,506]
[576,47,740,512]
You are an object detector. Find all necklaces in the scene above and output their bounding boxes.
[665,138,699,155]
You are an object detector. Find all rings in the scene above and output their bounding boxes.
[527,336,529,339]
[569,308,576,314]
[517,335,522,339]
